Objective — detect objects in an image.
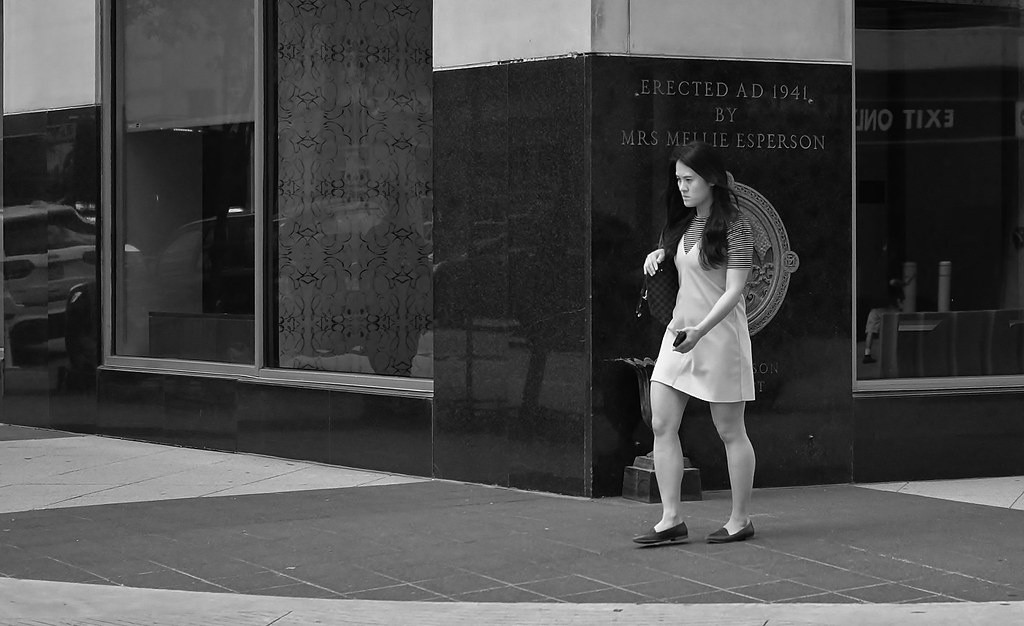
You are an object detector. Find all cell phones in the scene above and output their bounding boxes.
[673,331,686,347]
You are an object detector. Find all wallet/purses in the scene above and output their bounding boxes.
[673,331,686,348]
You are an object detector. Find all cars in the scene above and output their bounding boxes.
[155,210,257,315]
[0,200,147,377]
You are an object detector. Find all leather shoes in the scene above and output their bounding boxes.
[705,520,754,543]
[632,521,688,544]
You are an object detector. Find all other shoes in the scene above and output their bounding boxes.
[863,354,876,363]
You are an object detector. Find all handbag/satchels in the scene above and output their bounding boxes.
[634,252,679,325]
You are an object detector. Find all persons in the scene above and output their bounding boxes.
[632,139,757,545]
